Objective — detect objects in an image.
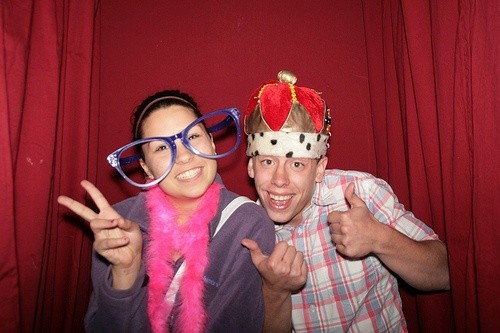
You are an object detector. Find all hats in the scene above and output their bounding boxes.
[245,68,332,159]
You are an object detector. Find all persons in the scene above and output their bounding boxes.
[239,69,450,333]
[56,89,275,333]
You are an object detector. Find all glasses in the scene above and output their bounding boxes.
[106,107,242,186]
